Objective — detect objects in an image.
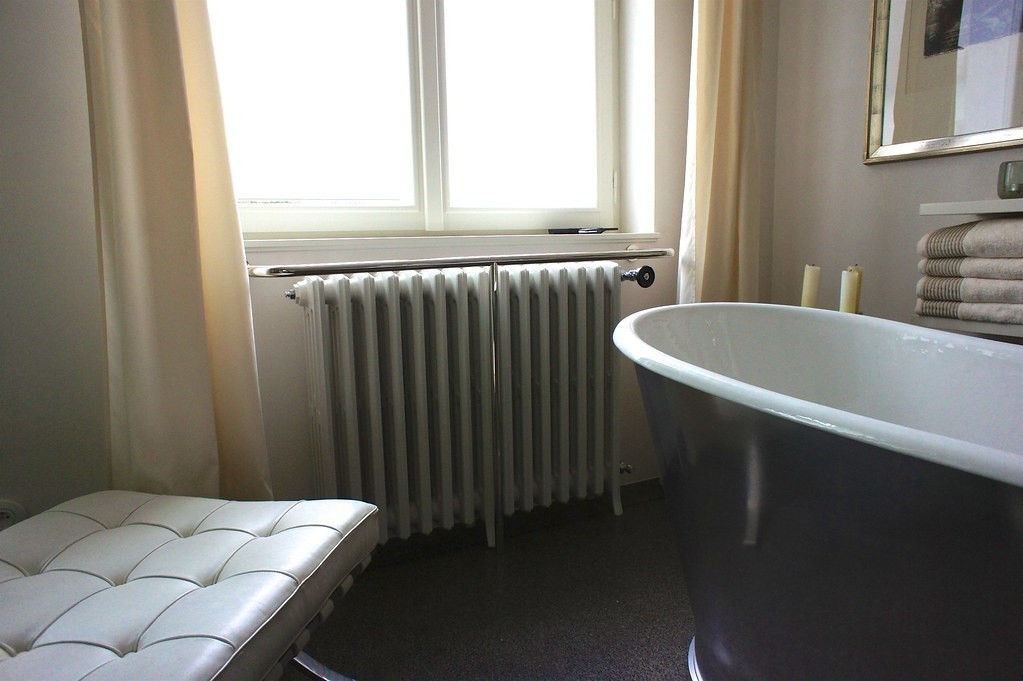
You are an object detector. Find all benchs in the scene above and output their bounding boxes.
[0,488,377,681]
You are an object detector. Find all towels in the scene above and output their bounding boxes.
[916,215,1023,259]
[913,298,1023,323]
[914,276,1023,303]
[917,255,1023,280]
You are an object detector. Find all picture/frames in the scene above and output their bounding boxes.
[861,1,1023,165]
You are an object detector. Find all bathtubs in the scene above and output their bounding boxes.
[611,299,1023,681]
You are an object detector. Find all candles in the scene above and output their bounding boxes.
[800,264,820,307]
[848,266,861,314]
[840,271,857,314]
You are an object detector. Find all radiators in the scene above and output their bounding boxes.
[285,261,622,550]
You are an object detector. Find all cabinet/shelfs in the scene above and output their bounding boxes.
[910,198,1023,338]
